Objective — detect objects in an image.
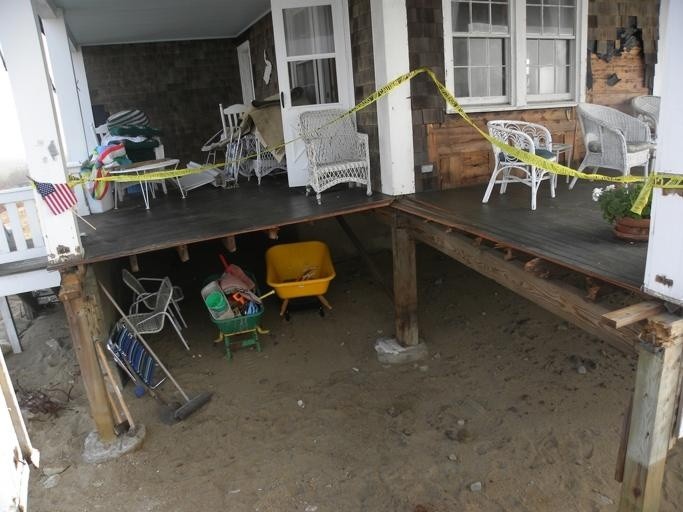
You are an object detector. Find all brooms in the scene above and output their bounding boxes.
[97,278,214,421]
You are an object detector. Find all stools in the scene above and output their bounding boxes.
[523,142,575,189]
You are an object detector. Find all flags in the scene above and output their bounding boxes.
[33,180,78,217]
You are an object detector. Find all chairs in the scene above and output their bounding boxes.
[567,101,651,196]
[218,100,253,141]
[233,129,287,187]
[88,122,167,195]
[119,276,192,352]
[298,108,373,206]
[480,120,556,211]
[630,93,661,152]
[121,269,185,332]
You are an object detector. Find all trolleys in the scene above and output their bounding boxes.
[202,270,270,360]
[265,240,335,320]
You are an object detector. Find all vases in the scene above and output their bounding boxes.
[610,217,650,243]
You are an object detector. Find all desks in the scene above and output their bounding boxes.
[111,158,183,211]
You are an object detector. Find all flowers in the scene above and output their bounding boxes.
[587,180,653,226]
